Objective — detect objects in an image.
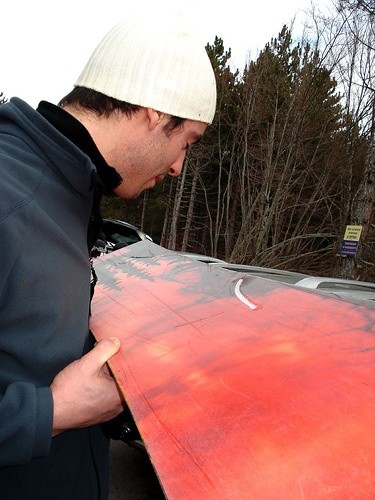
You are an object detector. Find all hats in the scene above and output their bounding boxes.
[73,10,218,125]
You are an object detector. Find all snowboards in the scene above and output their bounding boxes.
[89,237,375,500]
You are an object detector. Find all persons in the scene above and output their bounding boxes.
[0,7,217,500]
